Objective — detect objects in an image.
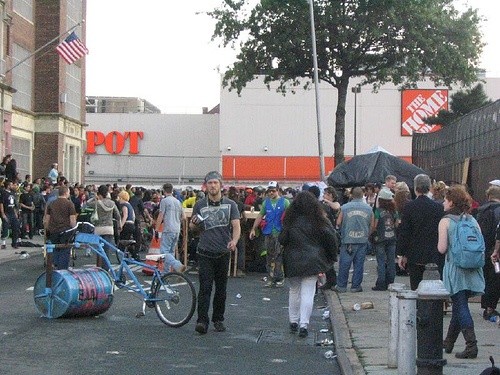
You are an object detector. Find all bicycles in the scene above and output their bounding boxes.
[33,221,197,329]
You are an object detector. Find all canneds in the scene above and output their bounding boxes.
[494,262,500,273]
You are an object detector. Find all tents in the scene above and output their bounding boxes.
[325,145,433,189]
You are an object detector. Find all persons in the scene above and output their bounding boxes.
[189,171,241,335]
[438,186,484,359]
[0,155,482,291]
[477,179,500,322]
[276,192,341,339]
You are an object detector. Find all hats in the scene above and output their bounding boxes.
[378,189,394,200]
[267,181,279,189]
[395,182,410,195]
[489,180,500,187]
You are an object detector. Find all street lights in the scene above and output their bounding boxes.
[352,86,361,154]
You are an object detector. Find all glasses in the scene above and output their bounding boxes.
[268,190,277,192]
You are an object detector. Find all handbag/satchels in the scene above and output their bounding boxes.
[90,201,99,223]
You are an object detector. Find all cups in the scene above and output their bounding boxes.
[324,351,333,358]
[260,277,267,281]
[352,304,360,311]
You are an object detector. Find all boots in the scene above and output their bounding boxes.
[455,327,478,359]
[443,320,461,354]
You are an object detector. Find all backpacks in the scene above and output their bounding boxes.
[442,210,485,268]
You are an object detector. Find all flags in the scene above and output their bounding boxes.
[58,31,89,65]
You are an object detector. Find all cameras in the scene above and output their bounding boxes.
[195,214,209,224]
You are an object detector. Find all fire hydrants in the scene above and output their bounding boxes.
[414,262,453,375]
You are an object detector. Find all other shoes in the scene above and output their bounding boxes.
[175,264,186,282]
[331,286,346,292]
[289,322,299,333]
[318,284,334,290]
[21,236,33,239]
[264,279,285,288]
[299,328,308,337]
[216,318,226,331]
[195,319,210,331]
[371,286,387,290]
[236,270,245,277]
[483,311,500,322]
[350,287,362,292]
[1,240,6,249]
